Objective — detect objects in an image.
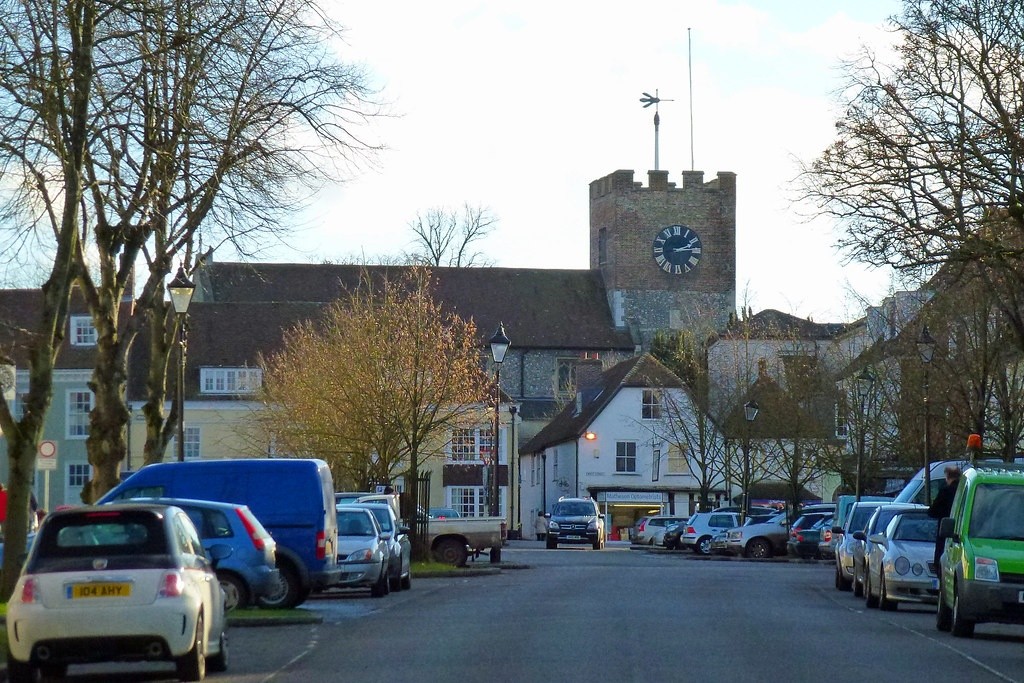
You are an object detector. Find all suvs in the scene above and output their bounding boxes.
[784,511,835,559]
[544,496,606,550]
[632,502,836,558]
[0,497,279,612]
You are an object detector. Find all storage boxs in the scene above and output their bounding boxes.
[610,525,629,541]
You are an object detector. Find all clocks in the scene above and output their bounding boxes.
[652,224,702,274]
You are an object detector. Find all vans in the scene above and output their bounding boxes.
[90,459,337,607]
[937,467,1024,637]
[892,459,1024,507]
[831,495,894,552]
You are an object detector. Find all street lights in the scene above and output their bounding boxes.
[741,399,758,525]
[487,321,511,564]
[854,366,875,501]
[915,326,935,505]
[165,261,197,459]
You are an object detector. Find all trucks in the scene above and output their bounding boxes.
[352,496,508,566]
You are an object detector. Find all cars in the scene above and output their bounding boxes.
[5,503,234,683]
[336,504,413,592]
[335,507,392,597]
[428,508,459,518]
[835,501,931,600]
[867,513,939,610]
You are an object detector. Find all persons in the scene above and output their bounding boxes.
[0,483,38,543]
[534,511,547,541]
[928,466,963,577]
[776,501,785,514]
